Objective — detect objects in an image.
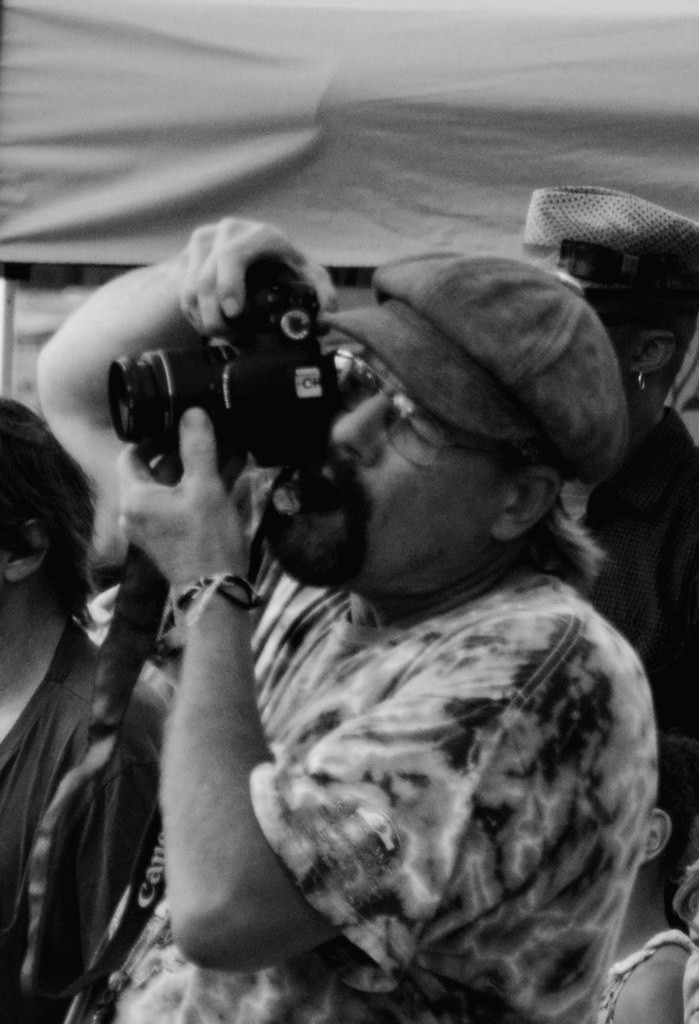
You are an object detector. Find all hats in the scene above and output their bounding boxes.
[319,250,627,483]
[524,185,699,321]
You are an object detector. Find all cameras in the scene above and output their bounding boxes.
[105,255,345,476]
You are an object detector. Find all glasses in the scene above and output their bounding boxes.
[329,348,477,468]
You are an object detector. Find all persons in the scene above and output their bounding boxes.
[32,216,658,1023]
[0,397,177,1024]
[523,185,699,739]
[598,731,699,1024]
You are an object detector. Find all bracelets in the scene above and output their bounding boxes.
[177,570,259,611]
